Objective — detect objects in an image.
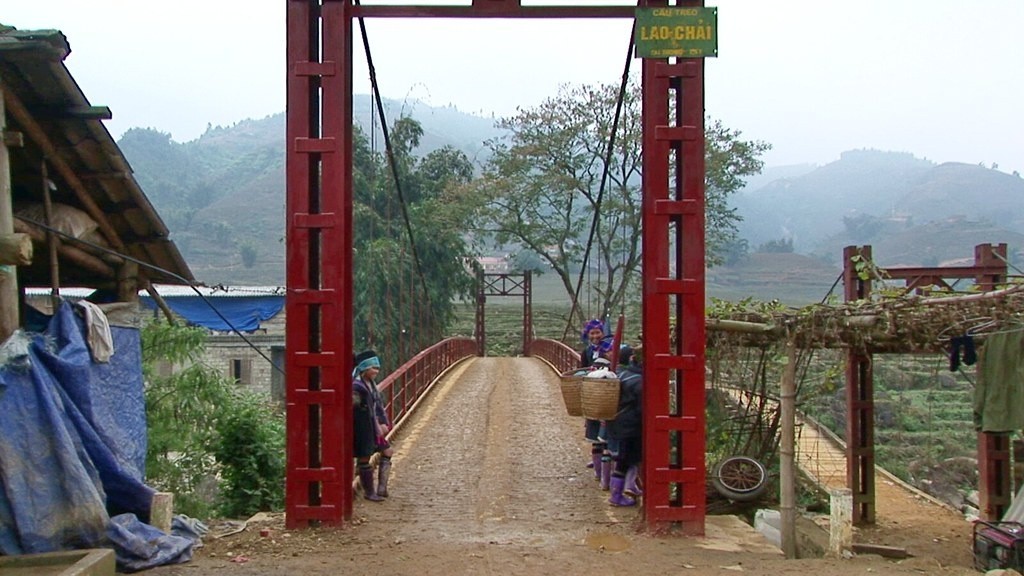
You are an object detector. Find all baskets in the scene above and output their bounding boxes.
[560,367,592,416]
[581,376,622,420]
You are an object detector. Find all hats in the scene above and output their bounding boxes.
[351,350,380,379]
[582,318,605,340]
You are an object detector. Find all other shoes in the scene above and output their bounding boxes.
[586,460,594,468]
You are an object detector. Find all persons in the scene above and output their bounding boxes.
[581,319,643,506]
[352,350,393,501]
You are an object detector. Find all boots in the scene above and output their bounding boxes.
[359,466,385,501]
[608,476,635,506]
[377,461,393,497]
[592,452,603,480]
[599,461,612,490]
[624,464,642,496]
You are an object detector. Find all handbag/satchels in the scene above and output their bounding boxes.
[587,367,618,379]
